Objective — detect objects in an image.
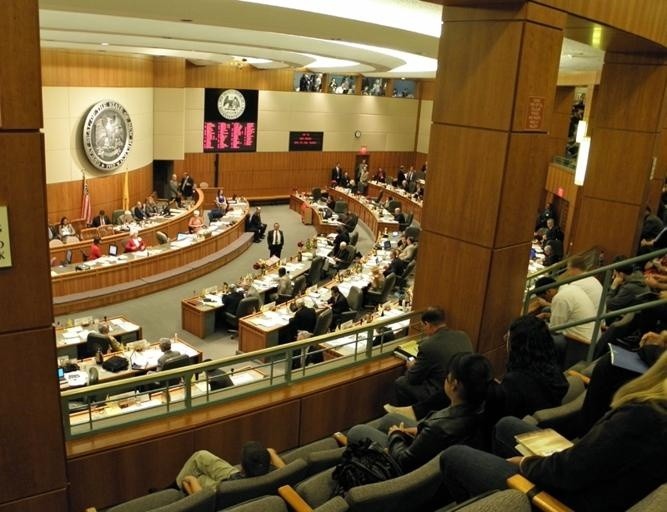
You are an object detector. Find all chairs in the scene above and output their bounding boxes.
[82,349,667,512]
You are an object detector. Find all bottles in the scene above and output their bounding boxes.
[298,252,302,262]
[599,250,605,267]
[174,333,178,343]
[96,348,103,364]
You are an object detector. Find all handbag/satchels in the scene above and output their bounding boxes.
[331,436,402,488]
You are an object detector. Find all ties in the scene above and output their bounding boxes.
[275,230,277,245]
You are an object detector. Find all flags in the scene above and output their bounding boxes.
[122,170,130,210]
[81,174,94,224]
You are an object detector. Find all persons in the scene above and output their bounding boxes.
[148,440,280,496]
[92,210,112,228]
[180,171,194,200]
[168,174,180,202]
[348,201,665,510]
[300,72,415,97]
[188,210,204,232]
[156,337,182,372]
[202,358,234,390]
[221,156,428,364]
[98,322,122,352]
[88,236,103,260]
[57,216,75,236]
[124,228,146,253]
[116,195,160,225]
[215,189,227,210]
